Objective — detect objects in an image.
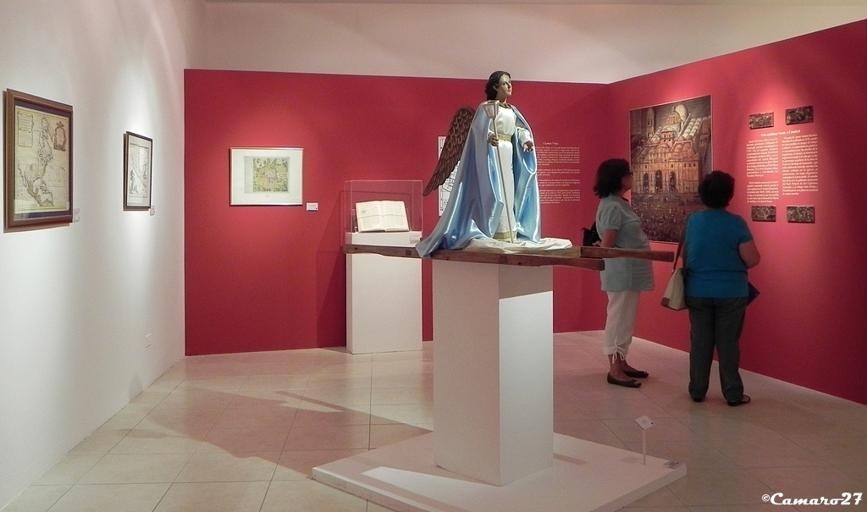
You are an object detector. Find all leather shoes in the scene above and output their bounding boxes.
[622,369,648,378]
[607,371,642,388]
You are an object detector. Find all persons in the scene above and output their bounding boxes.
[415,68,539,255]
[591,158,654,389]
[679,168,760,407]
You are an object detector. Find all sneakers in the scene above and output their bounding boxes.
[690,392,704,402]
[728,394,751,406]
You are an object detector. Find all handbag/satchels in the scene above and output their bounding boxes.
[582,221,601,247]
[660,267,686,311]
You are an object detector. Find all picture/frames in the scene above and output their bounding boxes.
[229,146,304,206]
[4,88,73,234]
[123,130,153,211]
